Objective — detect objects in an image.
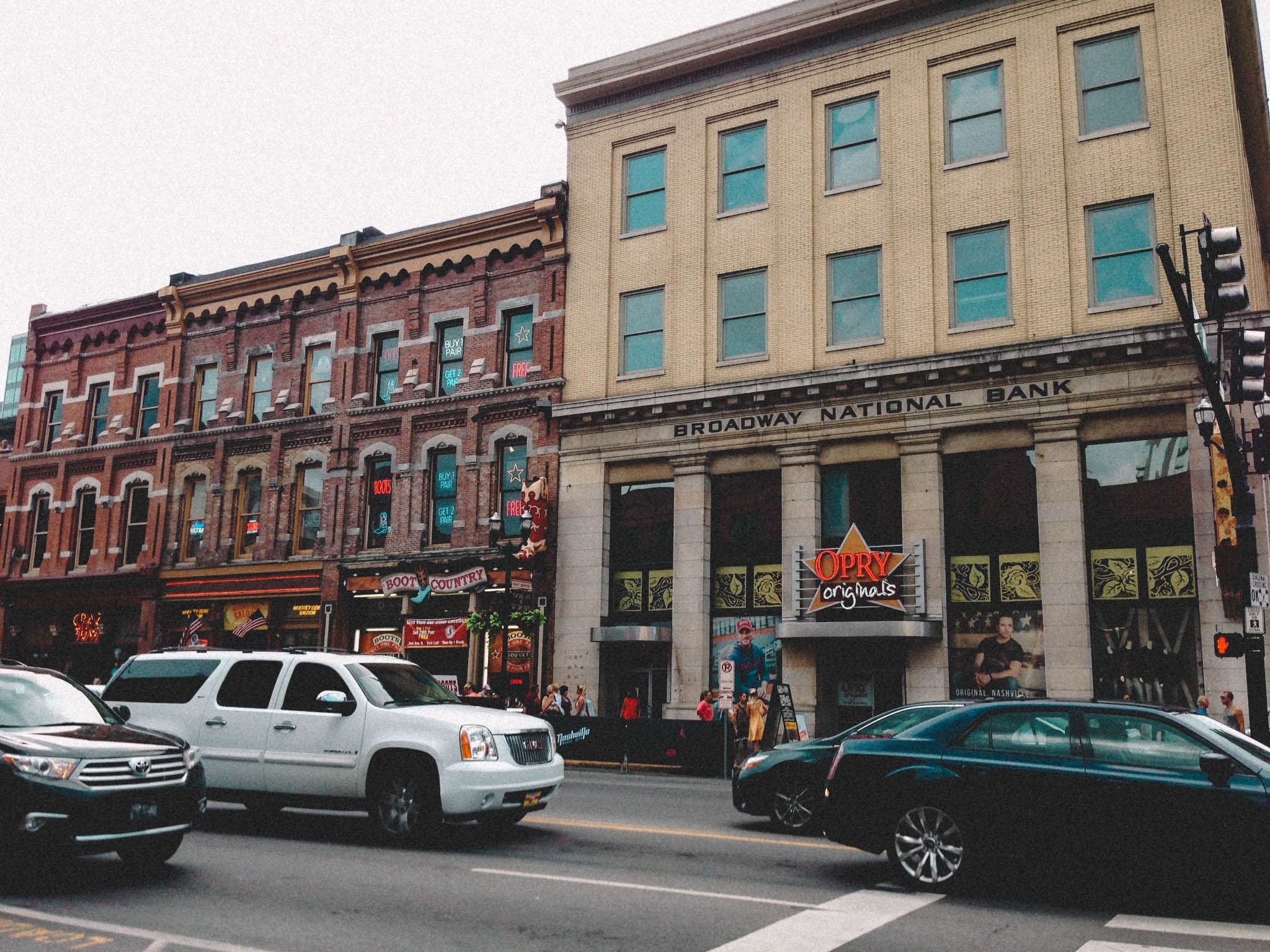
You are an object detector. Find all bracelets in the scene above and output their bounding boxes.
[989,673,993,680]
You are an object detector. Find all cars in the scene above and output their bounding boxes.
[819,698,1270,917]
[729,700,1100,836]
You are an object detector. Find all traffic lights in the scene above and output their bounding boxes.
[1212,633,1244,657]
[1199,213,1248,320]
[1233,326,1267,401]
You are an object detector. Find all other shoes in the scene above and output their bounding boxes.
[734,765,739,770]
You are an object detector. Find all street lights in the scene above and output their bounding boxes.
[1195,392,1269,746]
[488,510,532,707]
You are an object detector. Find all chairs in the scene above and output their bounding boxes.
[1046,726,1126,761]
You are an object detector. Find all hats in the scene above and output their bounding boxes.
[484,684,491,690]
[737,618,753,633]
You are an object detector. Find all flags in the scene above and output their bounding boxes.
[233,609,269,638]
[181,609,203,650]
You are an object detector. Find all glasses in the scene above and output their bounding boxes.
[1220,696,1227,700]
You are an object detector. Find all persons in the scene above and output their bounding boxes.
[463,682,596,717]
[1196,691,1246,735]
[725,618,769,703]
[621,687,641,719]
[954,613,1024,698]
[696,688,768,768]
[1096,640,1179,706]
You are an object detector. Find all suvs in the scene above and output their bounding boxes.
[100,647,565,843]
[0,657,208,883]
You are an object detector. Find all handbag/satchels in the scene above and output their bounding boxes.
[545,703,561,716]
